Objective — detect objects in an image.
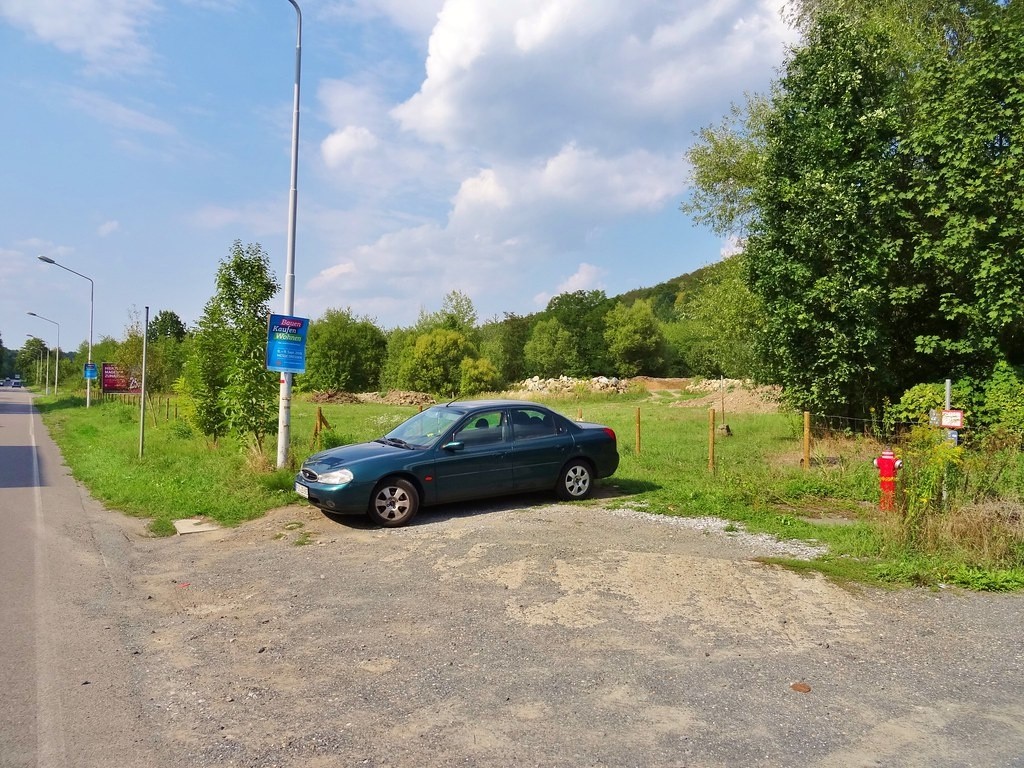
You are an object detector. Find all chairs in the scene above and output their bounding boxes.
[474,418,488,430]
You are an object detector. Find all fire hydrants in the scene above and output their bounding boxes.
[873,450,903,511]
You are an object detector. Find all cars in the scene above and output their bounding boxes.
[294,399,619,528]
[12,381,21,388]
[0,380,4,385]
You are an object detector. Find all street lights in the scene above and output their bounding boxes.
[26,334,49,387]
[27,312,59,395]
[38,256,93,408]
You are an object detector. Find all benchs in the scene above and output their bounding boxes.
[514,412,559,431]
